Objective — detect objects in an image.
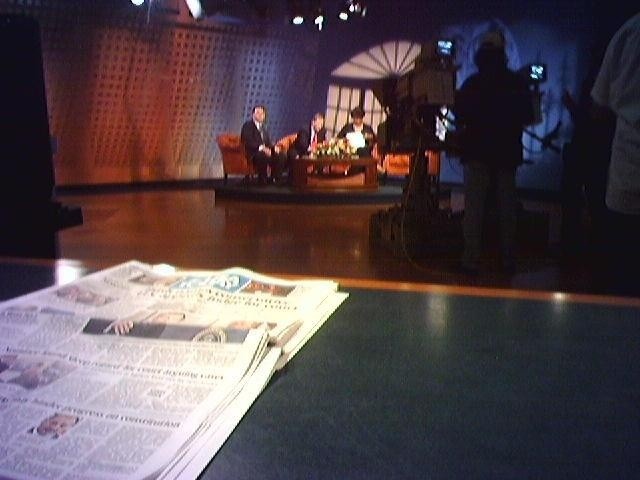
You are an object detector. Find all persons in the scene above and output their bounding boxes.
[335,107,378,158]
[591,10,639,298]
[286,112,333,181]
[241,106,281,185]
[454,36,543,274]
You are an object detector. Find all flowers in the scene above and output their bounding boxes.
[312,137,356,158]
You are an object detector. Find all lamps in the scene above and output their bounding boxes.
[289,0,364,29]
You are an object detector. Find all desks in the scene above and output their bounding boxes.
[3,252,640,478]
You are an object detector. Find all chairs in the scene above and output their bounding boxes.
[383,149,440,185]
[216,133,280,187]
